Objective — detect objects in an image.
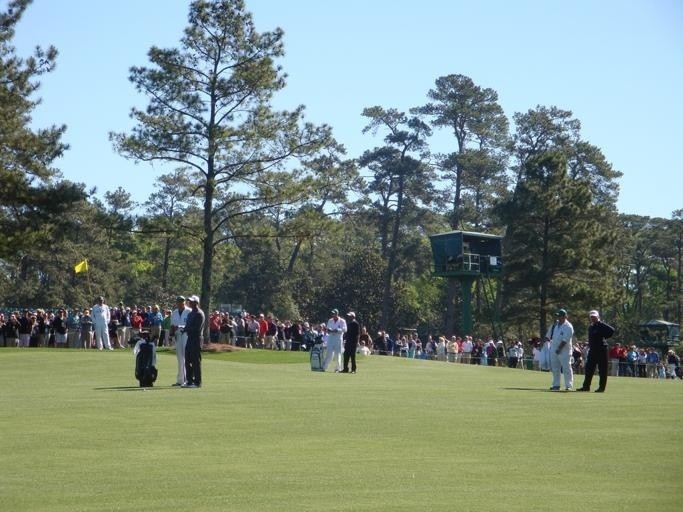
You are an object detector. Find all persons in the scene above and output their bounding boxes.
[543,312,560,340]
[471,334,552,372]
[319,309,347,373]
[1,295,175,349]
[208,309,325,351]
[545,309,574,392]
[572,339,682,379]
[179,295,206,387]
[338,311,359,374]
[356,326,373,354]
[169,295,192,387]
[376,328,682,378]
[446,253,463,272]
[378,329,472,364]
[576,309,615,392]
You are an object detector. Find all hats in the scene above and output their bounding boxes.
[187,295,199,303]
[331,308,339,315]
[590,310,598,317]
[553,309,567,317]
[346,312,355,317]
[174,295,185,302]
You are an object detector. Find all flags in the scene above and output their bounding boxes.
[74,259,88,273]
